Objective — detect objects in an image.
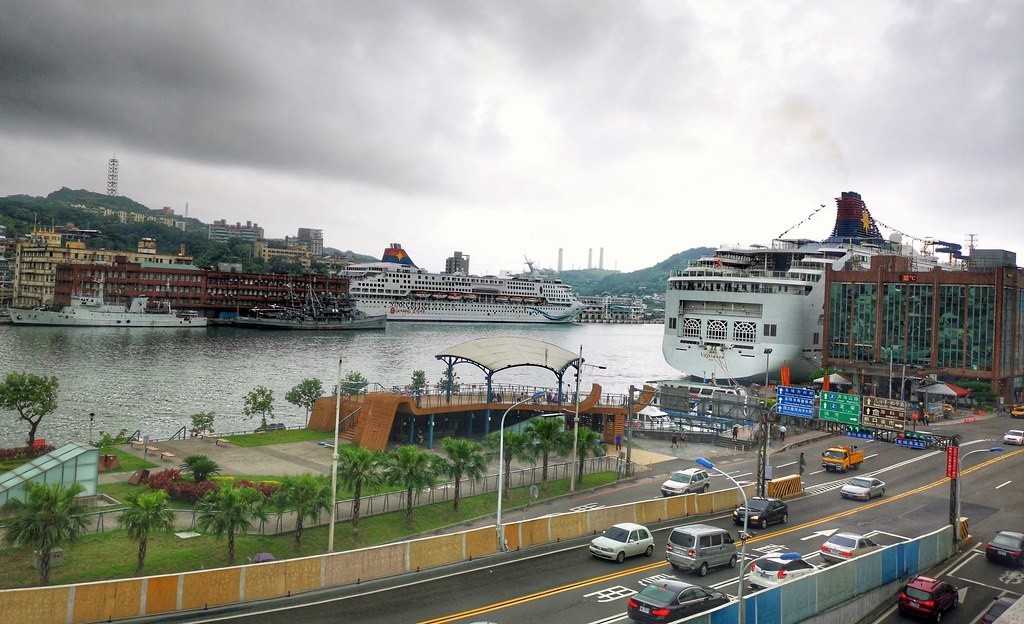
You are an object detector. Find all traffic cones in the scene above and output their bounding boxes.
[640,460,652,472]
[707,446,736,460]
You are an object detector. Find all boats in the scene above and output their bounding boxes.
[447,293,463,300]
[414,291,432,298]
[510,296,523,304]
[7,279,208,327]
[464,293,478,301]
[525,297,538,304]
[230,274,388,331]
[432,292,449,299]
[633,379,764,433]
[495,295,509,303]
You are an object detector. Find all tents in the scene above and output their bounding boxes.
[813,371,853,392]
[915,383,971,411]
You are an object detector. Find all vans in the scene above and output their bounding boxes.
[665,524,738,578]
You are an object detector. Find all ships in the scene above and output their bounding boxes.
[662,191,978,387]
[336,261,590,324]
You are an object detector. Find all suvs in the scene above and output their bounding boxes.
[898,574,959,624]
[661,466,710,497]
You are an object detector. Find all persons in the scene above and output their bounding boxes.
[616,434,622,450]
[417,428,422,437]
[799,452,807,476]
[534,397,542,405]
[553,393,558,401]
[491,391,502,402]
[547,393,552,401]
[679,428,688,447]
[779,425,786,442]
[915,402,926,422]
[998,404,1002,413]
[671,431,678,448]
[732,427,738,441]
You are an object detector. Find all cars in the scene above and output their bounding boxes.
[818,531,882,563]
[626,578,731,624]
[986,531,1024,567]
[840,477,886,502]
[980,597,1017,624]
[1011,406,1024,419]
[748,551,819,589]
[733,497,788,529]
[1003,429,1024,446]
[588,523,655,564]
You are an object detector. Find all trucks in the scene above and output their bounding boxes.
[821,445,864,473]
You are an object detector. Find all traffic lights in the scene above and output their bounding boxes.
[846,425,873,434]
[903,431,927,440]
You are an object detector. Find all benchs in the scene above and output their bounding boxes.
[130,440,143,447]
[159,451,175,459]
[215,438,230,446]
[203,434,213,439]
[144,446,158,452]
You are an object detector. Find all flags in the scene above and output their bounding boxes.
[717,259,722,268]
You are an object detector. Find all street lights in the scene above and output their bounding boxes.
[695,456,802,602]
[497,391,546,526]
[315,361,368,553]
[957,447,1005,541]
[763,348,773,408]
[568,344,607,493]
[89,411,96,444]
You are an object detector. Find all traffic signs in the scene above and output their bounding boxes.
[819,390,860,425]
[862,396,906,432]
[777,386,815,419]
